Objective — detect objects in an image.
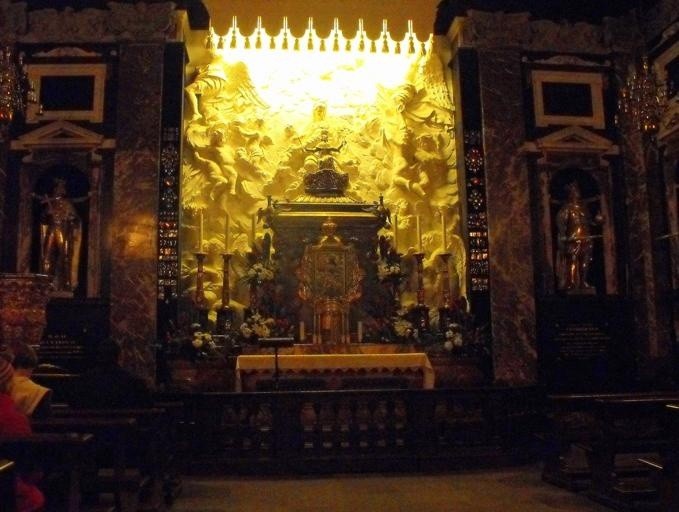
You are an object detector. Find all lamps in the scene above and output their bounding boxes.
[613,56,672,132]
[1,46,44,123]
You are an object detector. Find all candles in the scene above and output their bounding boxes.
[225,213,230,254]
[358,320,363,342]
[251,213,256,246]
[417,215,421,252]
[299,321,305,340]
[394,214,398,250]
[441,213,446,250]
[199,212,204,253]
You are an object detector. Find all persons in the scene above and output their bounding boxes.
[0,354,45,510]
[552,180,602,291]
[29,177,84,291]
[10,352,50,431]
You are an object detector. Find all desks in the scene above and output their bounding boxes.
[235,345,435,391]
